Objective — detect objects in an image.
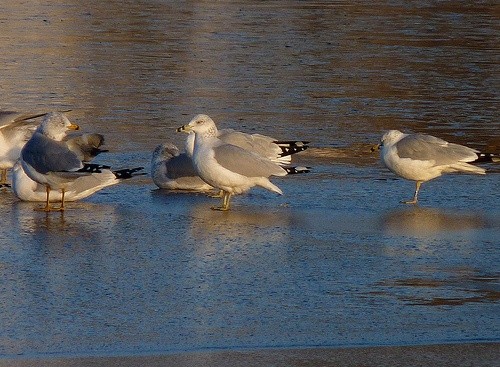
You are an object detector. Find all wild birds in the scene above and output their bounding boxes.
[370,129,499,205]
[0,109,149,212]
[151,114,313,211]
